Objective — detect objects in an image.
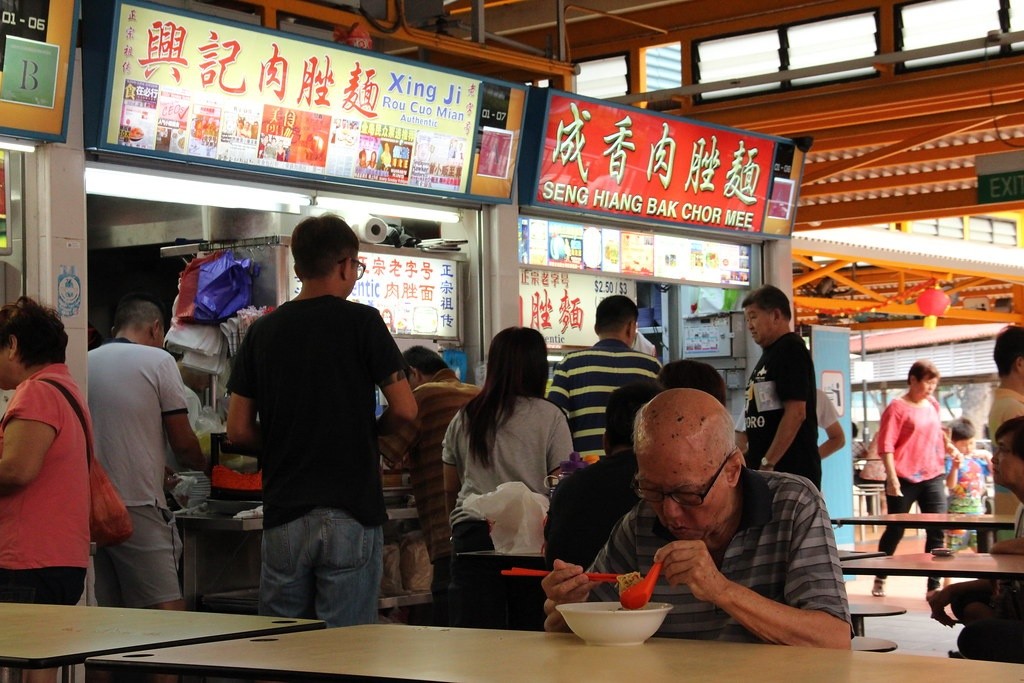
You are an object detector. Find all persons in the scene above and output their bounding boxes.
[0,295,95,683]
[542,388,856,649]
[735,388,845,459]
[381,347,480,627]
[943,419,993,588]
[852,423,887,513]
[225,215,418,628]
[871,360,959,603]
[88,299,207,683]
[441,327,573,631]
[547,293,662,461]
[988,325,1024,516]
[928,417,1024,663]
[544,360,726,573]
[741,285,822,493]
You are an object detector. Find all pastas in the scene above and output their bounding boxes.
[617,572,646,611]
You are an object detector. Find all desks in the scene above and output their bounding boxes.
[830,511,1019,554]
[76,623,1024,683]
[0,599,336,683]
[840,551,1024,580]
[836,548,885,561]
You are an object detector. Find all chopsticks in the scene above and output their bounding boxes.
[501,567,623,581]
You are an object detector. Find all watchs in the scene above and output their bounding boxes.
[761,457,774,468]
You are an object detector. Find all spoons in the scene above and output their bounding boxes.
[620,562,660,609]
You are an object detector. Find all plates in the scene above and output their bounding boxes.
[180,472,211,499]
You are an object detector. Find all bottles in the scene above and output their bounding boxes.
[550,452,587,500]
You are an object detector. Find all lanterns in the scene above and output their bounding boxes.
[918,284,952,328]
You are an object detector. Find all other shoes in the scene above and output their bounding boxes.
[926,588,942,601]
[872,578,887,597]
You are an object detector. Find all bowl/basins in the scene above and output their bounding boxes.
[555,603,674,646]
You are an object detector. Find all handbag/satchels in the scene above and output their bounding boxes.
[463,481,551,553]
[85,457,133,543]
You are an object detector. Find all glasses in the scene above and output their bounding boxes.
[337,258,366,281]
[628,447,738,506]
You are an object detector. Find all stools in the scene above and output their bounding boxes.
[850,604,909,634]
[858,483,885,514]
[851,492,878,540]
[850,635,899,653]
[855,487,884,533]
